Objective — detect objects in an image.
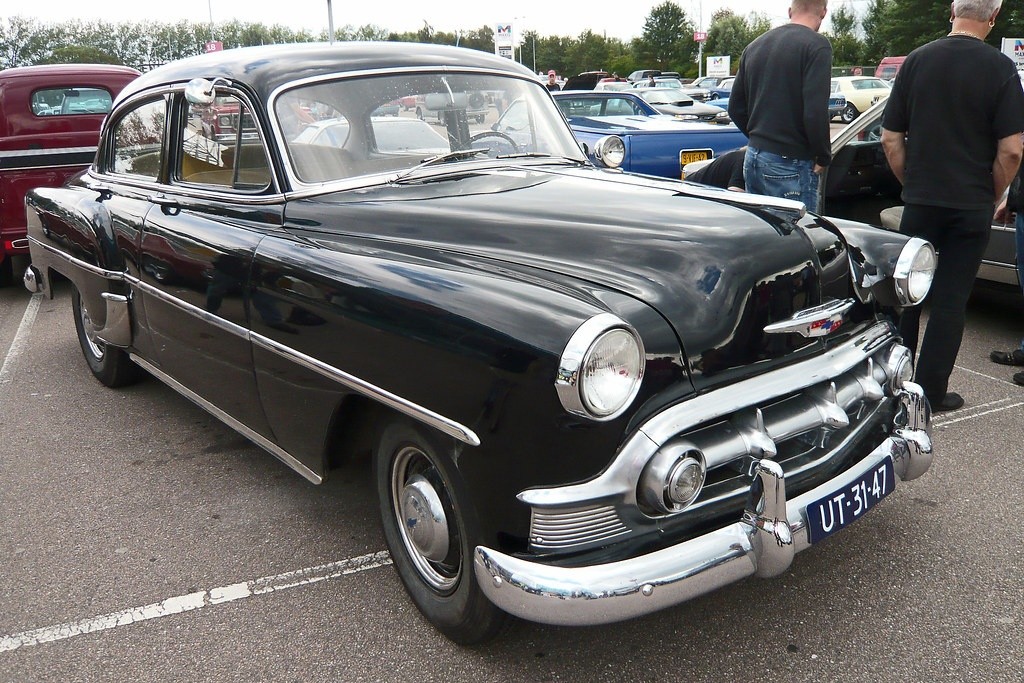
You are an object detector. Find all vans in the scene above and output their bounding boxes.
[577,71,610,82]
[874,56,907,86]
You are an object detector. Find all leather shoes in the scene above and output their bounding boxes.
[932,392,964,413]
[990,349,1024,366]
[1013,370,1024,387]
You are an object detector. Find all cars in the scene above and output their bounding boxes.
[332,102,401,117]
[537,75,566,91]
[14,40,939,648]
[0,63,150,287]
[467,90,751,181]
[588,86,732,126]
[678,89,1023,287]
[289,116,490,163]
[593,69,737,104]
[829,75,892,124]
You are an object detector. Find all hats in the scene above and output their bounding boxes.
[548,70,556,81]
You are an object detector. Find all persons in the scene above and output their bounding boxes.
[727,0,833,219]
[545,69,561,92]
[277,95,317,146]
[683,146,749,193]
[990,142,1024,389]
[877,0,1024,412]
[648,74,655,88]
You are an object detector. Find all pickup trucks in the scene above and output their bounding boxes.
[705,93,847,122]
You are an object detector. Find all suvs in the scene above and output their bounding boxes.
[414,91,490,125]
